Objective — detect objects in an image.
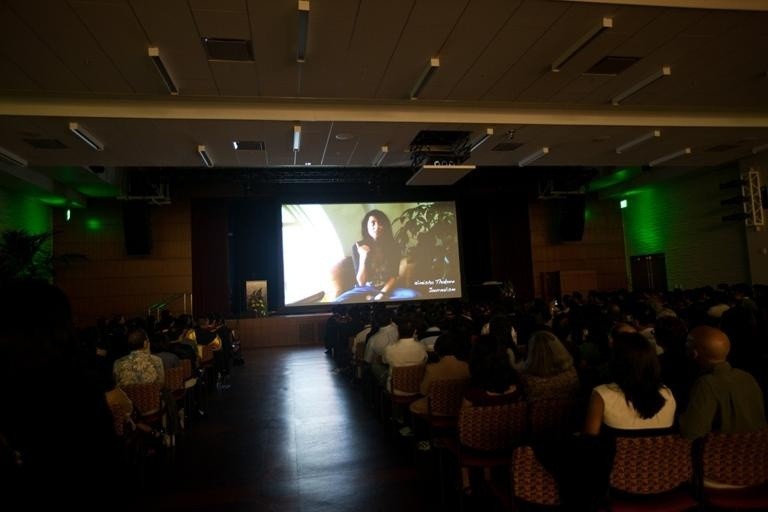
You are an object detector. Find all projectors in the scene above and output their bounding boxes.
[410,137,472,175]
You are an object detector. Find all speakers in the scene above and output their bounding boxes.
[122,202,153,257]
[545,198,587,243]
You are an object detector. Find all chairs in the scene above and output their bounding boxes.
[452,396,529,486]
[512,444,558,502]
[606,436,696,505]
[701,430,765,504]
[104,323,242,456]
[342,301,583,437]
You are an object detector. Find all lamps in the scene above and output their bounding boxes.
[472,129,694,169]
[410,57,441,102]
[295,0,310,65]
[1,118,215,171]
[149,44,180,97]
[293,125,388,167]
[548,15,672,106]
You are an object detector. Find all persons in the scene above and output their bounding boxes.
[104,310,244,448]
[326,281,765,466]
[334,209,424,302]
